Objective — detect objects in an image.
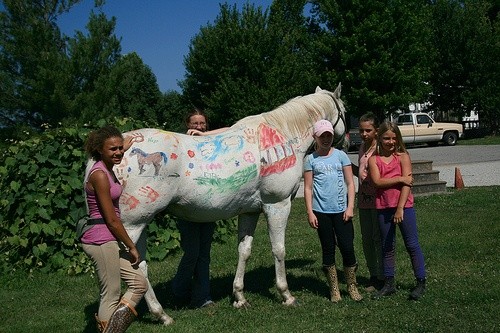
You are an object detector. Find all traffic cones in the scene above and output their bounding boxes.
[452,166,468,190]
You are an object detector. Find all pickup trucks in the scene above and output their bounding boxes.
[349,112,463,151]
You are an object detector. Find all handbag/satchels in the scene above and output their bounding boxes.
[76,216,89,241]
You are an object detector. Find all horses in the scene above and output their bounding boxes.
[82,81,352,309]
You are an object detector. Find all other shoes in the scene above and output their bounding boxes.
[204,303,215,308]
[372,276,397,299]
[367,286,375,292]
[410,278,426,301]
[177,295,188,305]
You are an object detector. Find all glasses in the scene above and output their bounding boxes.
[189,122,206,126]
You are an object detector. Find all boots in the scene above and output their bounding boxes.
[322,263,343,303]
[95,299,138,333]
[344,264,363,302]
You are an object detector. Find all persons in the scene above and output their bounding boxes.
[368,121,426,300]
[169,110,215,309]
[351,112,384,293]
[304,120,363,303]
[81,125,149,333]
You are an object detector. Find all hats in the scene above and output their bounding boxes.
[313,120,334,137]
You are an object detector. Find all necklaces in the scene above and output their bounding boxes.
[365,143,375,158]
